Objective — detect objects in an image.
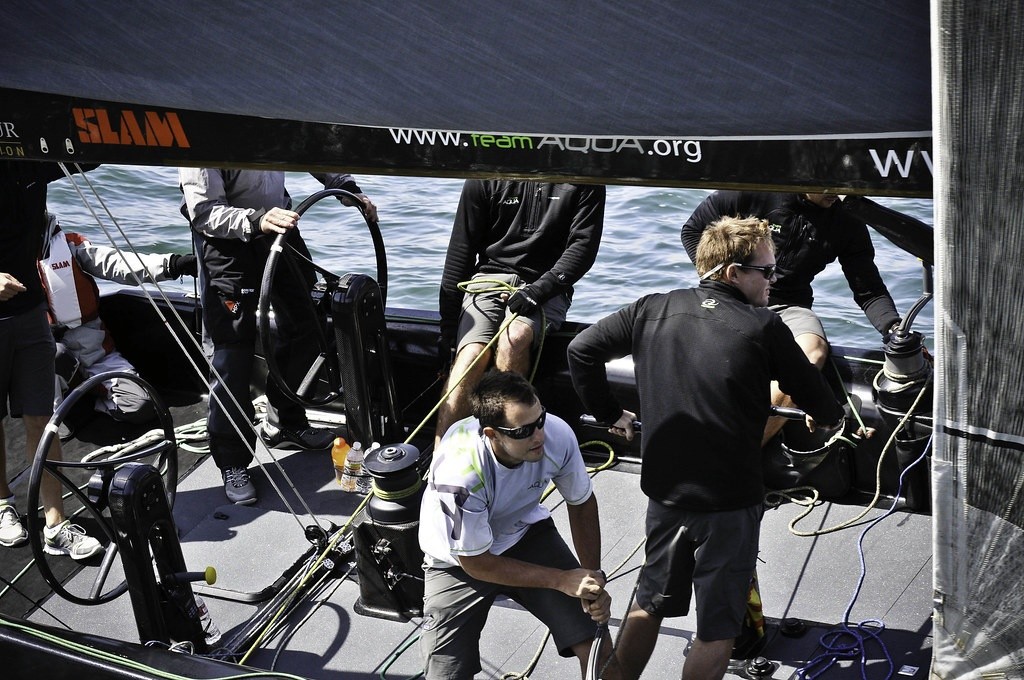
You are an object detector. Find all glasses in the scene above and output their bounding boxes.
[484,405,546,440]
[732,262,777,278]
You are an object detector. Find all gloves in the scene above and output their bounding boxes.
[506,283,544,316]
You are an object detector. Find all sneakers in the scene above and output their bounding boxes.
[260,415,337,451]
[221,467,258,505]
[57,413,73,439]
[0,495,28,547]
[43,520,106,561]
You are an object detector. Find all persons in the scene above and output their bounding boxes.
[38,205,199,444]
[432,179,606,455]
[681,188,925,447]
[567,215,845,680]
[1,159,105,562]
[178,165,378,504]
[418,370,623,680]
